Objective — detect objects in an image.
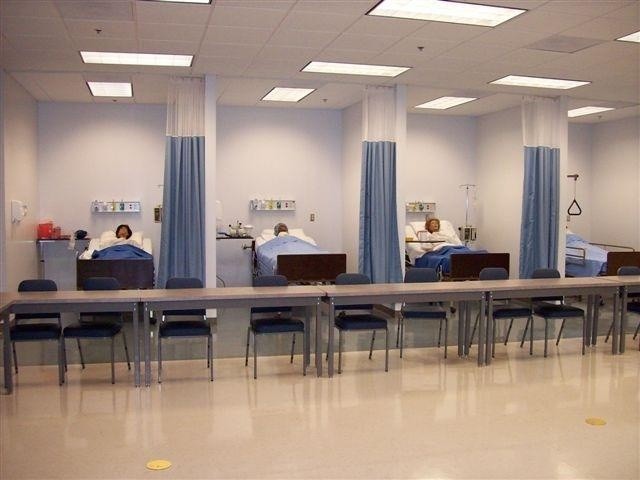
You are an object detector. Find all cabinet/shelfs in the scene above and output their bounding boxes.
[217,234,255,286]
[35,237,91,291]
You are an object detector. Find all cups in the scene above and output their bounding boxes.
[405,237,413,241]
[416,231,427,241]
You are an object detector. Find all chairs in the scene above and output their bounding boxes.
[0,266,640,394]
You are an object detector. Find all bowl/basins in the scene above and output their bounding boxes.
[60,235,71,240]
[458,226,477,241]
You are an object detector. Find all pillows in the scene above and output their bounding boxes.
[100,228,146,248]
[262,228,310,246]
[409,217,456,239]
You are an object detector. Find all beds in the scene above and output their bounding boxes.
[564,229,635,302]
[257,225,349,307]
[402,219,511,303]
[75,230,156,323]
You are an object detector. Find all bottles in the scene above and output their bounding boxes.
[50,226,65,239]
[229,223,248,238]
[405,200,430,212]
[252,199,298,210]
[91,200,139,212]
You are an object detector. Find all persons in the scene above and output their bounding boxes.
[274,222,289,236]
[425,218,454,249]
[112,225,137,246]
